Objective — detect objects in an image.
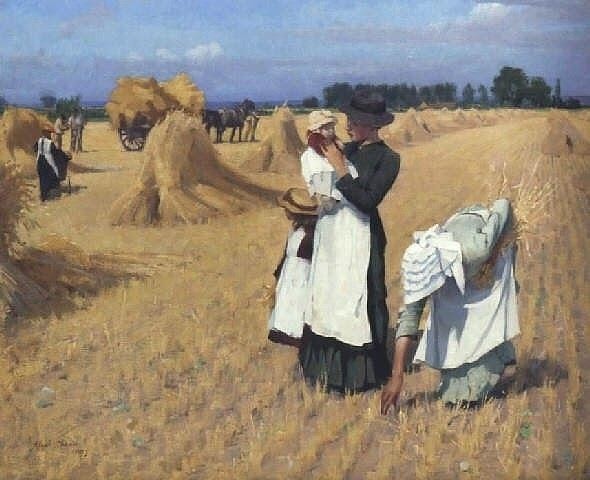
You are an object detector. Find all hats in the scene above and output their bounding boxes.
[275,188,319,216]
[307,110,337,134]
[336,91,394,128]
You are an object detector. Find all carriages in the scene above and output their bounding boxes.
[118,97,260,152]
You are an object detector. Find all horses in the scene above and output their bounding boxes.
[202,98,256,144]
